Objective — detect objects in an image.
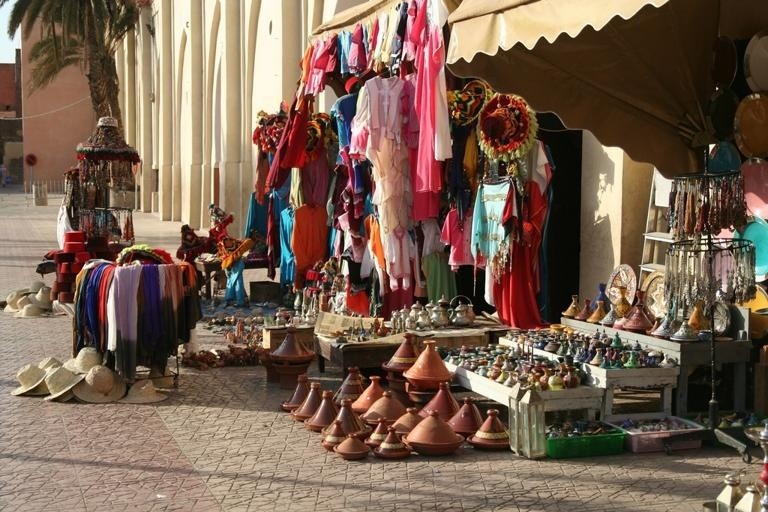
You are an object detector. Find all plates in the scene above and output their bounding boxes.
[639,270,670,323]
[604,265,637,311]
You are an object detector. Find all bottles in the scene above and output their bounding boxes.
[290,381,321,421]
[402,340,455,391]
[282,376,307,411]
[322,399,373,444]
[352,376,385,416]
[364,418,388,447]
[403,413,465,455]
[357,391,403,423]
[375,427,411,458]
[382,333,425,381]
[391,408,423,439]
[273,285,433,346]
[307,389,341,429]
[715,474,761,512]
[322,421,347,452]
[434,293,715,459]
[334,368,361,404]
[333,433,371,457]
[273,327,310,388]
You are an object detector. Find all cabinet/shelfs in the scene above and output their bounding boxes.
[194,256,280,300]
[318,330,518,383]
[445,306,751,461]
[633,160,676,291]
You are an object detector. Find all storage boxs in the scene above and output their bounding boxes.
[250,281,281,301]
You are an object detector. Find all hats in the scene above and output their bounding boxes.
[3,280,52,318]
[11,347,174,404]
[451,80,537,160]
